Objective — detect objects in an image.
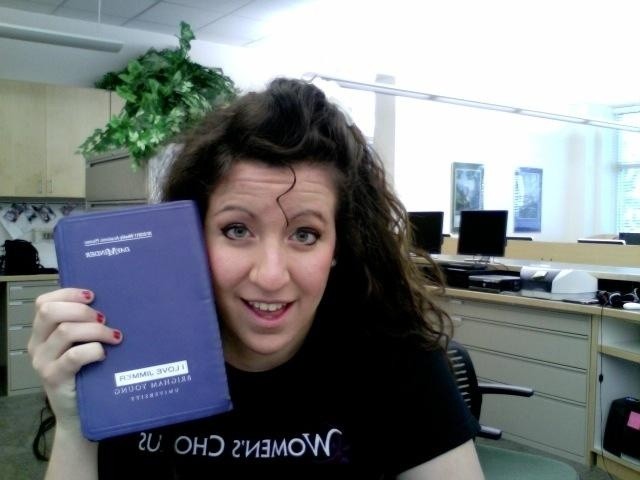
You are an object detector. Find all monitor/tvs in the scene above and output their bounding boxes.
[457,209,509,262]
[404,210,444,258]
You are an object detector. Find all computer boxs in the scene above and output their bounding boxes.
[468,274,521,294]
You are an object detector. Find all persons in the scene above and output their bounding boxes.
[22,74,489,480]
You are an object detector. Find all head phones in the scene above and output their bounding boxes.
[596,288,622,308]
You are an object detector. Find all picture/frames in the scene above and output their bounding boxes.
[450,160,545,236]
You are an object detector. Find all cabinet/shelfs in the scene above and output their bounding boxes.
[0,79,174,204]
[5,282,59,399]
[440,292,640,476]
